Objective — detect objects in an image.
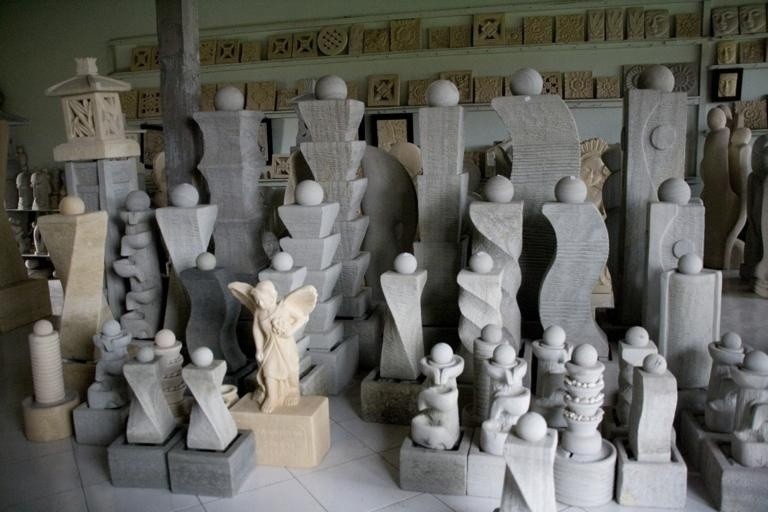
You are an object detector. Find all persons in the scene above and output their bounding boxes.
[716,6,737,34]
[227,278,318,415]
[646,12,667,38]
[718,42,733,62]
[719,73,736,95]
[738,3,767,32]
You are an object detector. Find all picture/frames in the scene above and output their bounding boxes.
[370,111,413,153]
[257,118,273,166]
[710,68,742,102]
[138,123,164,170]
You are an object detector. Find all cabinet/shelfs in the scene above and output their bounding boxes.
[5,207,63,280]
[704,1,768,140]
[104,1,703,193]
